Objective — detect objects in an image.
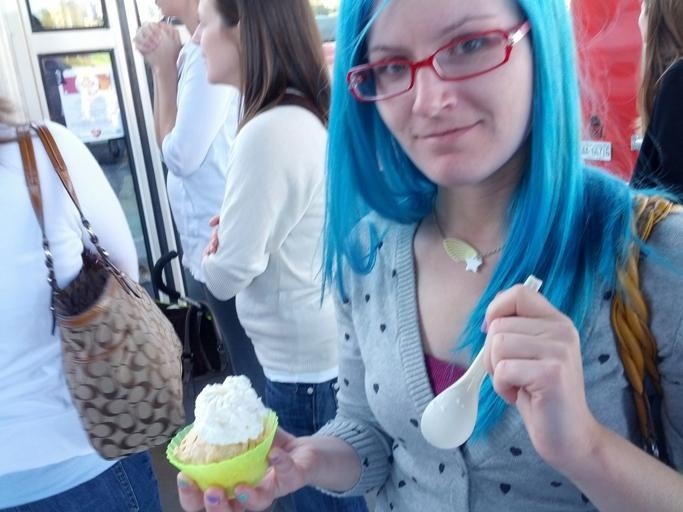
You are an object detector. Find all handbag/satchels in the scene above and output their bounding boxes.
[15,120,185,462]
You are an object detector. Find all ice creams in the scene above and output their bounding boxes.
[173,373,268,465]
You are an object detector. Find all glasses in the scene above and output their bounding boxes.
[347,17,531,107]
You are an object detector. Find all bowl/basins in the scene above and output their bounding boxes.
[165,408,282,500]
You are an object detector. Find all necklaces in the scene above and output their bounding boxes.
[431,193,506,272]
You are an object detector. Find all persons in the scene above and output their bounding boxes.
[191,0,368,512]
[0,98,183,512]
[630,0,683,204]
[133,0,267,407]
[177,0,683,512]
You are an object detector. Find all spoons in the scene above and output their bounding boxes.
[418,275,544,451]
